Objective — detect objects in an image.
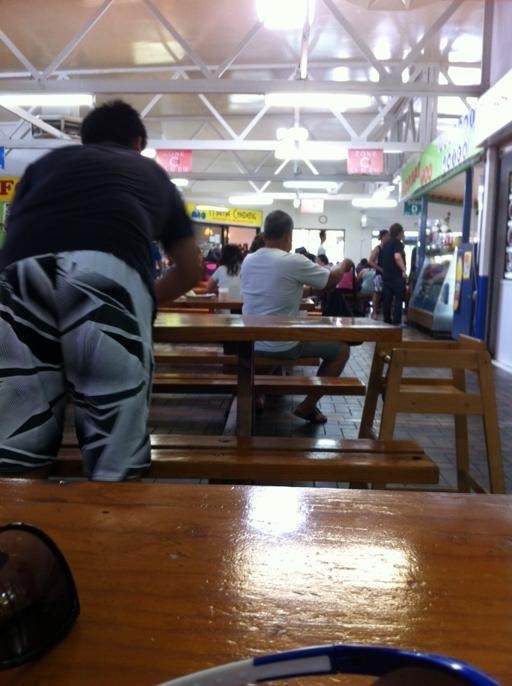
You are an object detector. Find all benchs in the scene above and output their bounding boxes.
[52,434,441,482]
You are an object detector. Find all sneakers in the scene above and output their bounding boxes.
[397,320,409,328]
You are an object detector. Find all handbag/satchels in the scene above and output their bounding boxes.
[317,262,370,346]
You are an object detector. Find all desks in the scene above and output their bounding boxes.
[149,291,404,442]
[0,476,510,686]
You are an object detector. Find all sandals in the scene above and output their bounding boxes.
[292,406,327,424]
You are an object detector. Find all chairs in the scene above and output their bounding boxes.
[351,332,509,494]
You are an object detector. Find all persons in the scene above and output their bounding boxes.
[242,243,248,255]
[0,96,206,489]
[368,223,408,326]
[318,230,326,255]
[251,232,266,254]
[238,210,354,424]
[207,243,242,314]
[295,247,375,294]
[204,247,221,276]
